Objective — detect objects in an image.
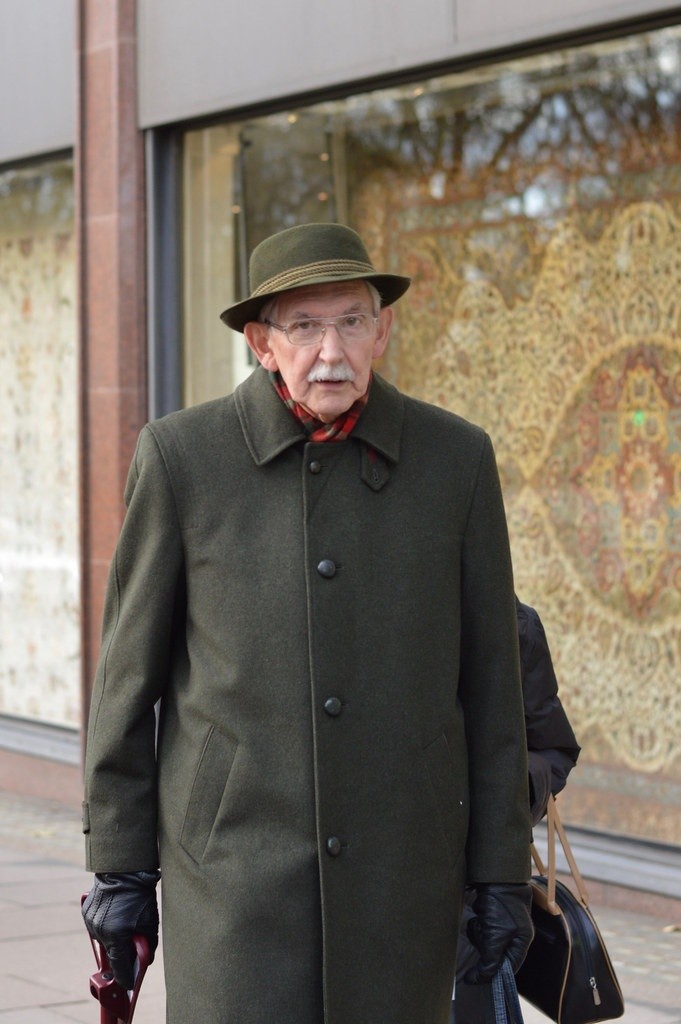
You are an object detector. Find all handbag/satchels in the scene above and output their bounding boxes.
[517,791,627,1024]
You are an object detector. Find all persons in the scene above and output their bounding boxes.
[81,224,582,1023]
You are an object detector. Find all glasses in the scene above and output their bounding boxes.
[265,314,380,342]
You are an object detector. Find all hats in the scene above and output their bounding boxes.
[218,223,410,334]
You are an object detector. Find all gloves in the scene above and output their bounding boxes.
[81,870,161,993]
[462,880,534,988]
